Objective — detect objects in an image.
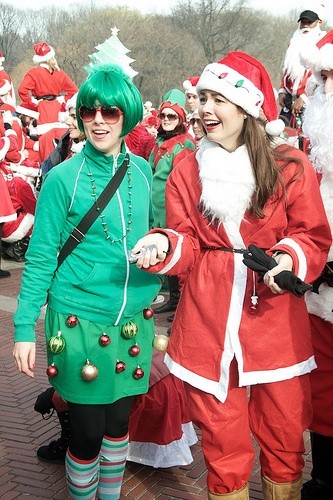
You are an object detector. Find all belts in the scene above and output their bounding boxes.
[202,245,270,254]
[35,95,57,100]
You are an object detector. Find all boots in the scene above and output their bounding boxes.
[37,413,71,465]
[261,476,303,500]
[207,481,250,500]
[168,315,175,321]
[300,431,333,500]
[0,236,30,263]
[155,276,179,313]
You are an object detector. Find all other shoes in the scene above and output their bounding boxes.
[0,269,10,278]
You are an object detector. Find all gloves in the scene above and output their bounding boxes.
[4,123,11,131]
[242,245,313,298]
[34,387,55,415]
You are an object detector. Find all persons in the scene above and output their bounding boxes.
[13,66,162,500]
[0,51,87,277]
[18,42,78,125]
[278,10,327,124]
[133,51,333,500]
[293,29,333,500]
[125,77,205,321]
[34,348,198,467]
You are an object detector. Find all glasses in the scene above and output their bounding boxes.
[157,112,181,121]
[78,105,124,124]
[190,118,202,126]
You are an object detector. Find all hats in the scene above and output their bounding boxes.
[299,29,333,72]
[65,91,78,110]
[32,42,55,64]
[0,76,11,96]
[298,10,318,23]
[158,89,187,123]
[184,76,200,97]
[195,52,286,136]
[15,97,40,120]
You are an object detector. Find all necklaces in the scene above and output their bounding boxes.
[83,153,133,243]
